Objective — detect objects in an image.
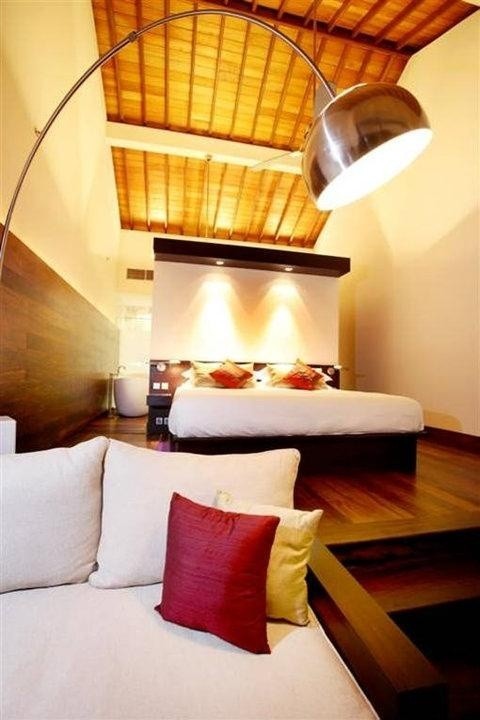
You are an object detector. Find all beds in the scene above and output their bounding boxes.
[166,360,427,478]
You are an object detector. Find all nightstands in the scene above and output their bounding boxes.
[145,394,170,439]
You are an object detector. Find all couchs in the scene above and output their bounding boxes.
[2,435,449,720]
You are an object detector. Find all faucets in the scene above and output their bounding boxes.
[117,365,126,374]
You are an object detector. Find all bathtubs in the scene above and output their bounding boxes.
[114,370,149,417]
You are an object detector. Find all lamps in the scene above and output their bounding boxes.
[1,7,435,275]
[328,365,342,375]
[157,357,181,372]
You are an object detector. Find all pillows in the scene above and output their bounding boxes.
[86,435,303,593]
[155,489,281,657]
[190,360,256,390]
[266,361,327,389]
[208,488,325,629]
[210,361,254,389]
[281,358,324,390]
[1,432,112,595]
